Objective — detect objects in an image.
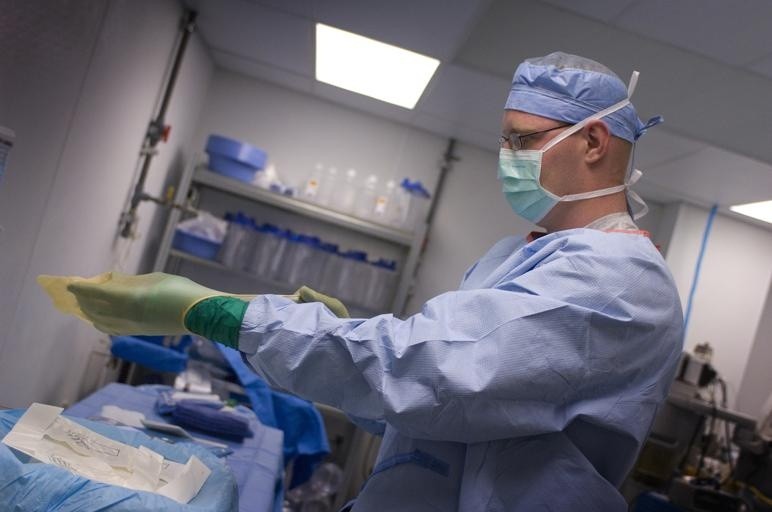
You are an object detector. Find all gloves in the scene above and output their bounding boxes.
[293,284,351,322]
[35,271,301,338]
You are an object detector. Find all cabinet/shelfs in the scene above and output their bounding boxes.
[102,157,428,502]
[618,392,758,500]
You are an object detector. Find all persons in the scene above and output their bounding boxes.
[67,49,690,512]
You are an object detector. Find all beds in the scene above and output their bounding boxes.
[57,384,287,512]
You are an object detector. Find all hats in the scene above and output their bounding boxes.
[498,72,650,225]
[503,53,664,143]
